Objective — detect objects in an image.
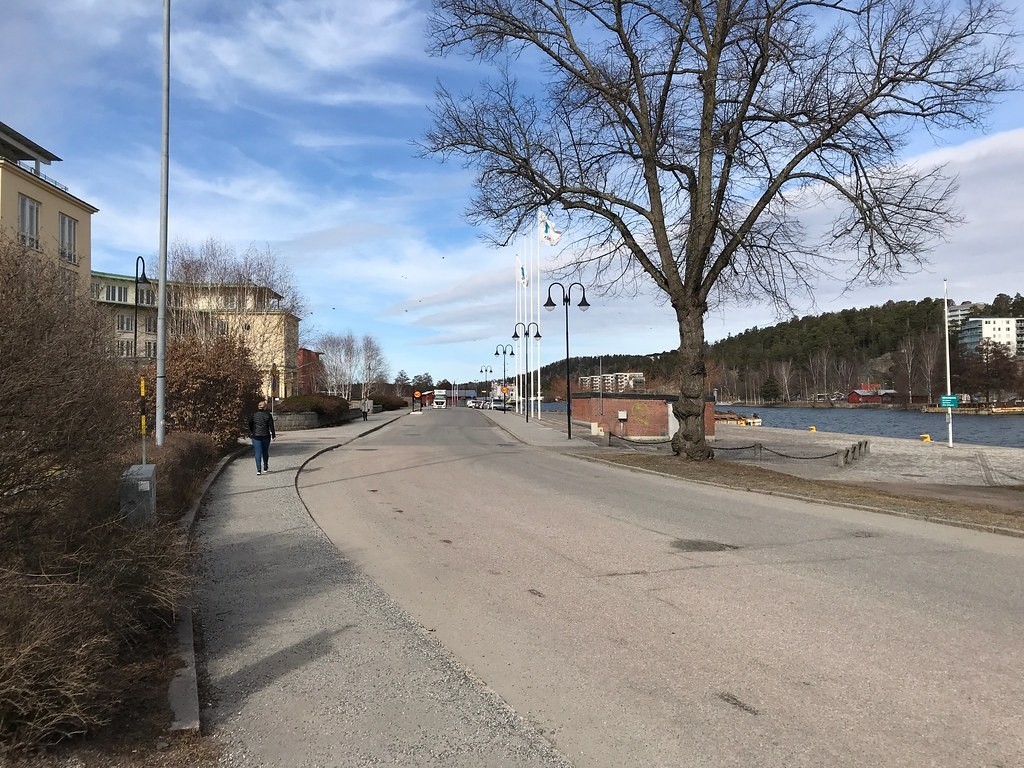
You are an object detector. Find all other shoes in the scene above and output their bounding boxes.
[257,472,260,475]
[263,470,267,472]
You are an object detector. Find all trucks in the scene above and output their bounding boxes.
[432,389,448,409]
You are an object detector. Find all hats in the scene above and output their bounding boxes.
[258,401,267,408]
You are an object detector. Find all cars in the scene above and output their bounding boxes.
[467,399,515,411]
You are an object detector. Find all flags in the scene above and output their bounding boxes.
[517,256,529,287]
[538,207,563,246]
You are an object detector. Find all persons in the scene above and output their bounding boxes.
[246,400,275,475]
[359,398,370,421]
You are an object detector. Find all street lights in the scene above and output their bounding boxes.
[542,282,591,439]
[493,343,515,414]
[480,365,493,397]
[134,255,152,355]
[511,321,542,422]
